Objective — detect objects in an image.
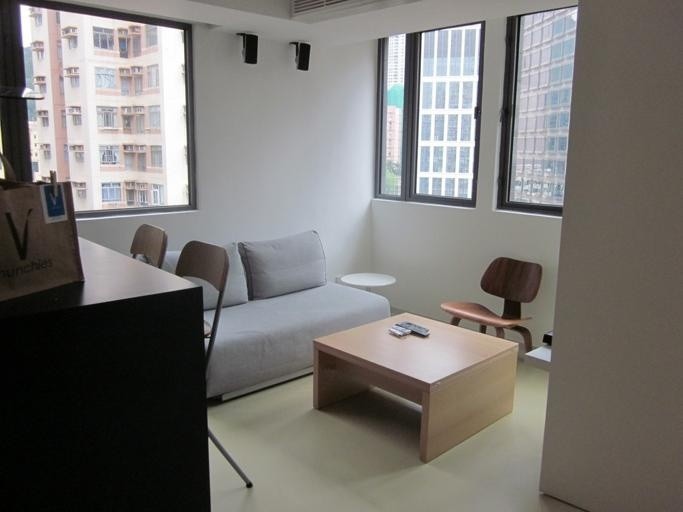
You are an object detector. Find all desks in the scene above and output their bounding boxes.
[0,237,211,512]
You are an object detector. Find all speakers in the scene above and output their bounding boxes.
[296,42,311,71]
[243,34,258,64]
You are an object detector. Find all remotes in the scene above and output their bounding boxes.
[395,321,431,336]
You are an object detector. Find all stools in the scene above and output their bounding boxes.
[340,273,396,292]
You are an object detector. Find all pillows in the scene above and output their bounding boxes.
[131,240,248,310]
[238,230,327,300]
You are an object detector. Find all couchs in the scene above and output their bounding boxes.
[135,229,391,401]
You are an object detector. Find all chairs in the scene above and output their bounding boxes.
[440,257,542,353]
[174,240,253,488]
[130,223,168,270]
